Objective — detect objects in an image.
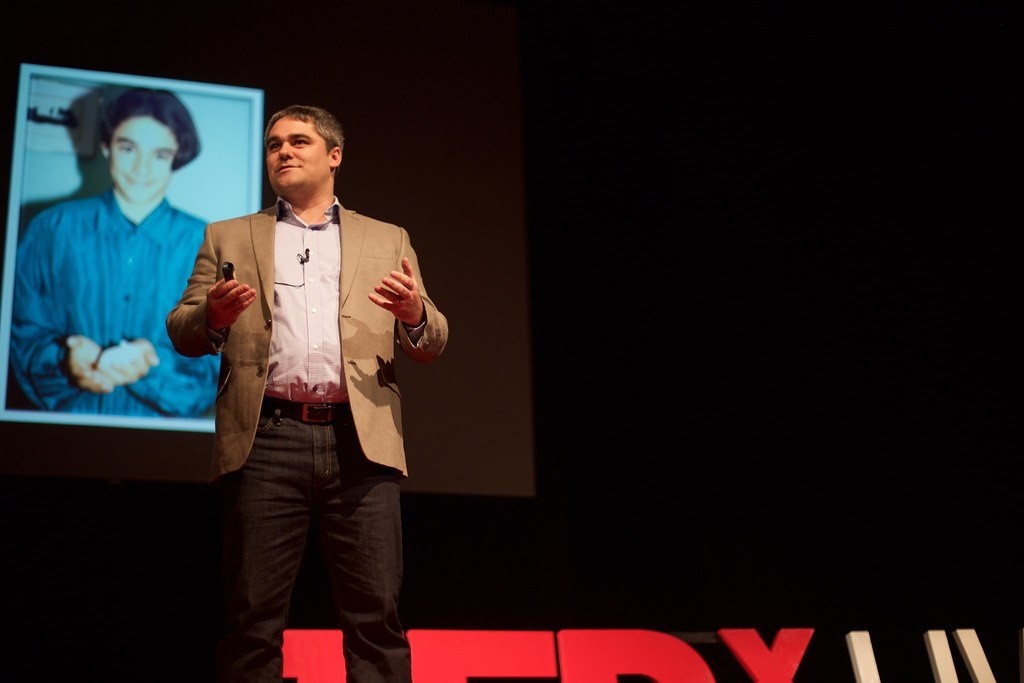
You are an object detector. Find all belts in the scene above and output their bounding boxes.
[260,392,354,424]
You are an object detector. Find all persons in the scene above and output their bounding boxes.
[164,102,451,683]
[10,84,244,419]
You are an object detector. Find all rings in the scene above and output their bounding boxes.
[237,307,241,314]
[392,298,401,305]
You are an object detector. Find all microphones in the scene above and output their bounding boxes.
[299,248,309,263]
